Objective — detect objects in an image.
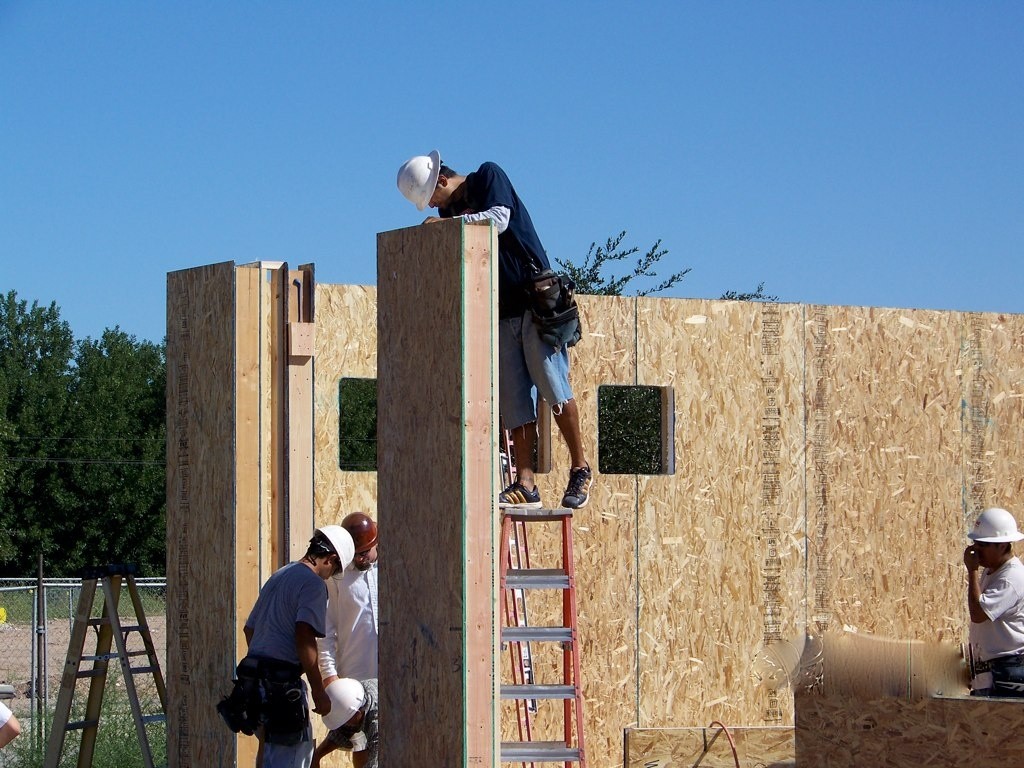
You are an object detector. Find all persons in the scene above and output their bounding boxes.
[236,524,356,768]
[956,506,1024,702]
[311,512,378,690]
[392,149,596,513]
[0,701,22,752]
[310,675,380,768]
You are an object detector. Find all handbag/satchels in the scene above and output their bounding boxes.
[532,268,582,347]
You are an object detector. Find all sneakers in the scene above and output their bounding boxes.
[498,484,543,509]
[562,461,594,509]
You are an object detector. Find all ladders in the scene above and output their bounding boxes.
[495,506,589,767]
[43,561,168,768]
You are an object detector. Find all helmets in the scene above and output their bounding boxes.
[396,150,440,210]
[340,512,382,551]
[320,677,366,730]
[314,525,354,580]
[967,507,1024,543]
